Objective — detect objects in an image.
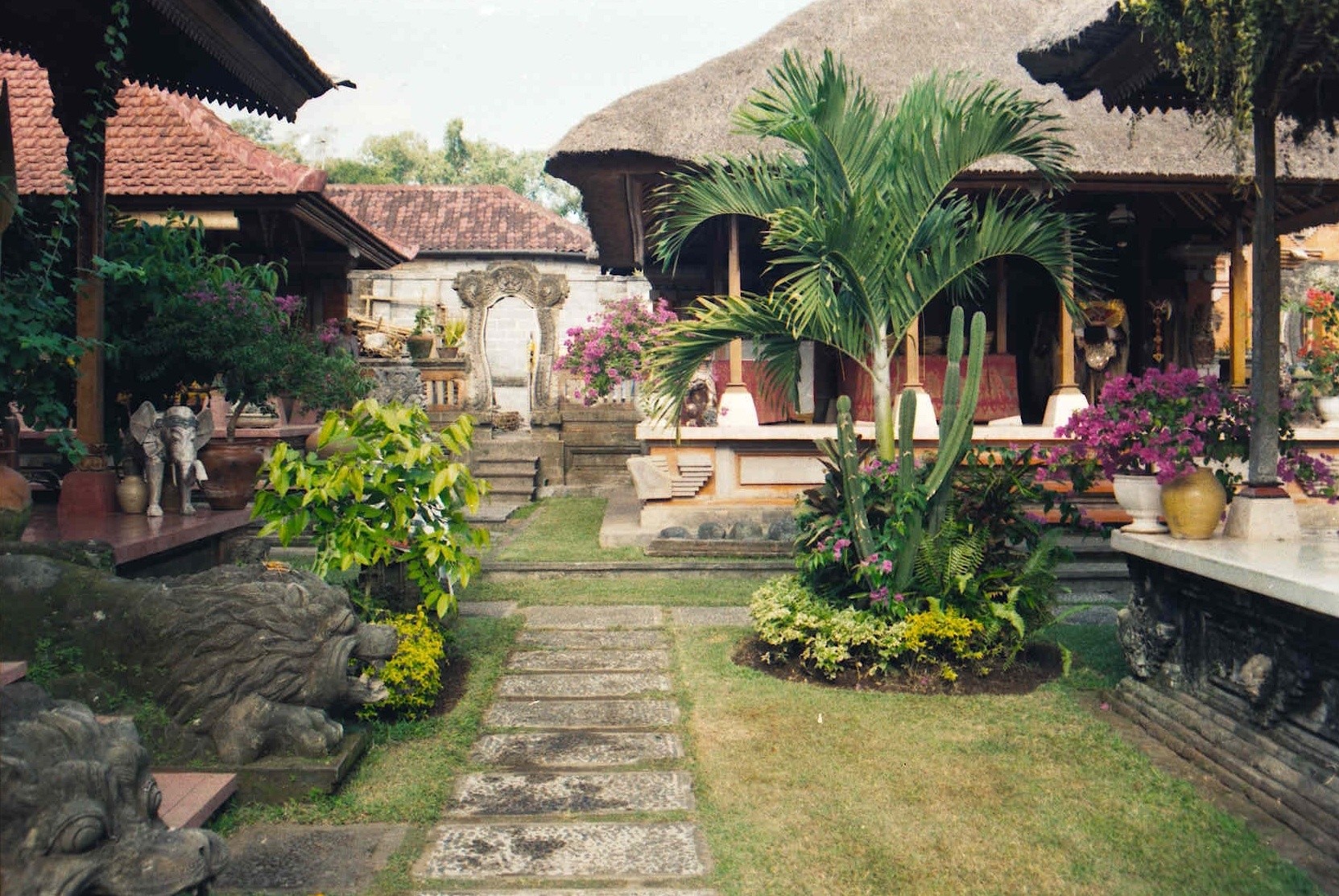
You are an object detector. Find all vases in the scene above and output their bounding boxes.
[304,426,359,457]
[633,389,679,426]
[1317,397,1339,428]
[1111,474,1169,533]
[198,441,264,511]
[1160,468,1228,539]
[116,476,149,514]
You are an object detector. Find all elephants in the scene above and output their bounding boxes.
[130,401,215,517]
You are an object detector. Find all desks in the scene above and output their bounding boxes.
[856,354,1020,422]
[712,361,788,425]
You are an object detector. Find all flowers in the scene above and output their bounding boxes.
[150,282,378,442]
[1053,284,1339,507]
[551,296,696,409]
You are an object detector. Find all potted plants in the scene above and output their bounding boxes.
[408,304,442,359]
[436,320,465,359]
